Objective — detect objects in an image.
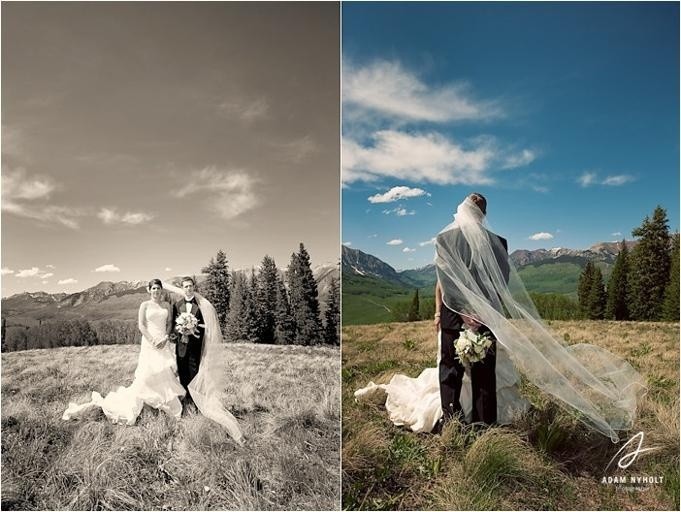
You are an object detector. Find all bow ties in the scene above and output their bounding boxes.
[183,298,192,305]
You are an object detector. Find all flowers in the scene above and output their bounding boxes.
[454,316,495,365]
[174,311,200,343]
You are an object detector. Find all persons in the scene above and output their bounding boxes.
[170,277,205,415]
[436,193,510,429]
[62,279,187,426]
[354,280,523,434]
